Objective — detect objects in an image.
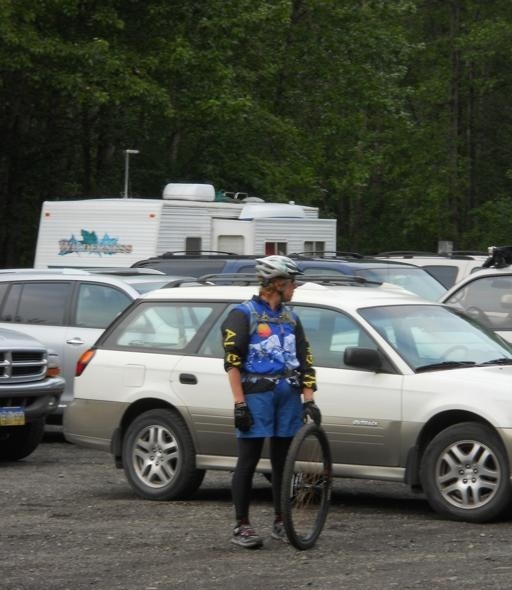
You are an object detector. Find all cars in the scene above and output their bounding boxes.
[1,328,67,463]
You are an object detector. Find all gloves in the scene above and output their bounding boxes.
[233,402,254,432]
[301,400,321,425]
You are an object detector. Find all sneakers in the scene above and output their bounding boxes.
[231,523,264,549]
[271,519,289,544]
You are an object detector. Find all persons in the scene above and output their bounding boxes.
[220,254,323,548]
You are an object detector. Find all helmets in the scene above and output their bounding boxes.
[255,255,304,281]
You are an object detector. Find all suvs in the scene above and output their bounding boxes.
[62,274,512,522]
[1,265,216,426]
[125,248,512,352]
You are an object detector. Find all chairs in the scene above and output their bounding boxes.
[357,319,420,374]
[304,327,336,367]
